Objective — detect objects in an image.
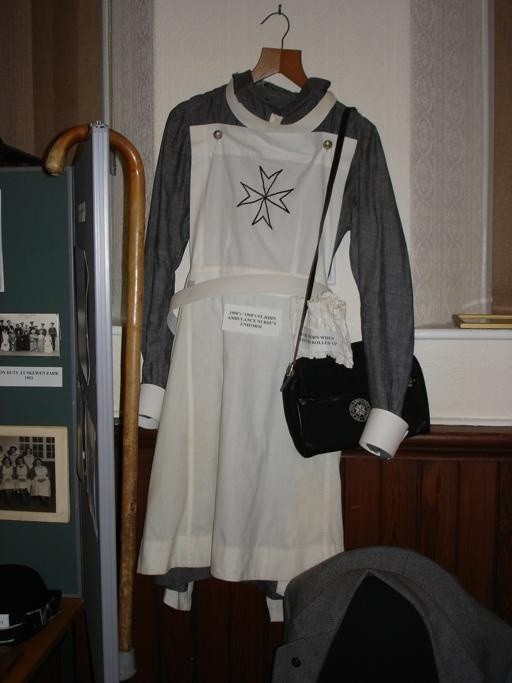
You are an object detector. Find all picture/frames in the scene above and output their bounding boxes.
[451,313,512,329]
[0,425,71,524]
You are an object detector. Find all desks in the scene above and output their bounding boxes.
[0,597,93,682]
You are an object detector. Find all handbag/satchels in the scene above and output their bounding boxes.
[281,340,431,458]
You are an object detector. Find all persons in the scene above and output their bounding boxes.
[1,319,58,353]
[0,445,53,504]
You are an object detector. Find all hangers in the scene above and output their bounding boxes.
[250,12,309,89]
